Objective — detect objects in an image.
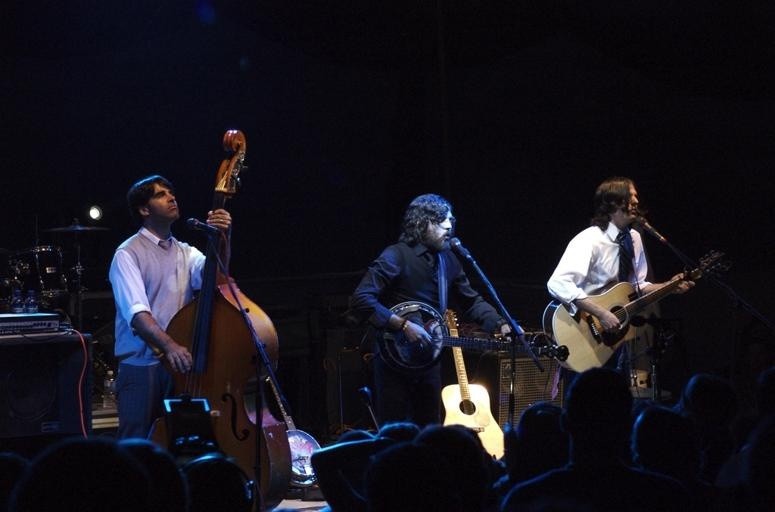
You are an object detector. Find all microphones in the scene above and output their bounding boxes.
[640,216,667,242]
[188,218,224,233]
[449,238,471,259]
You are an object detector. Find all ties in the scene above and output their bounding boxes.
[617,232,632,282]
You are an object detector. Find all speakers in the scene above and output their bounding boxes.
[441,345,563,432]
[0,333,92,438]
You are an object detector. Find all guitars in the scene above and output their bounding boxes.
[543,247,732,373]
[441,309,505,460]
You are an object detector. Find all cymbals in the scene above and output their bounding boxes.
[39,226,113,234]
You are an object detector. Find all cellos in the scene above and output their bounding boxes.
[147,130,291,512]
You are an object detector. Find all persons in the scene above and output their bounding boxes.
[108,174,235,443]
[2,435,255,512]
[354,192,513,432]
[333,366,774,512]
[546,176,695,399]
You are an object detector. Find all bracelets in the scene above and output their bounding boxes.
[400,319,412,331]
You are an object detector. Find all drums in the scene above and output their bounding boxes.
[8,244,73,299]
